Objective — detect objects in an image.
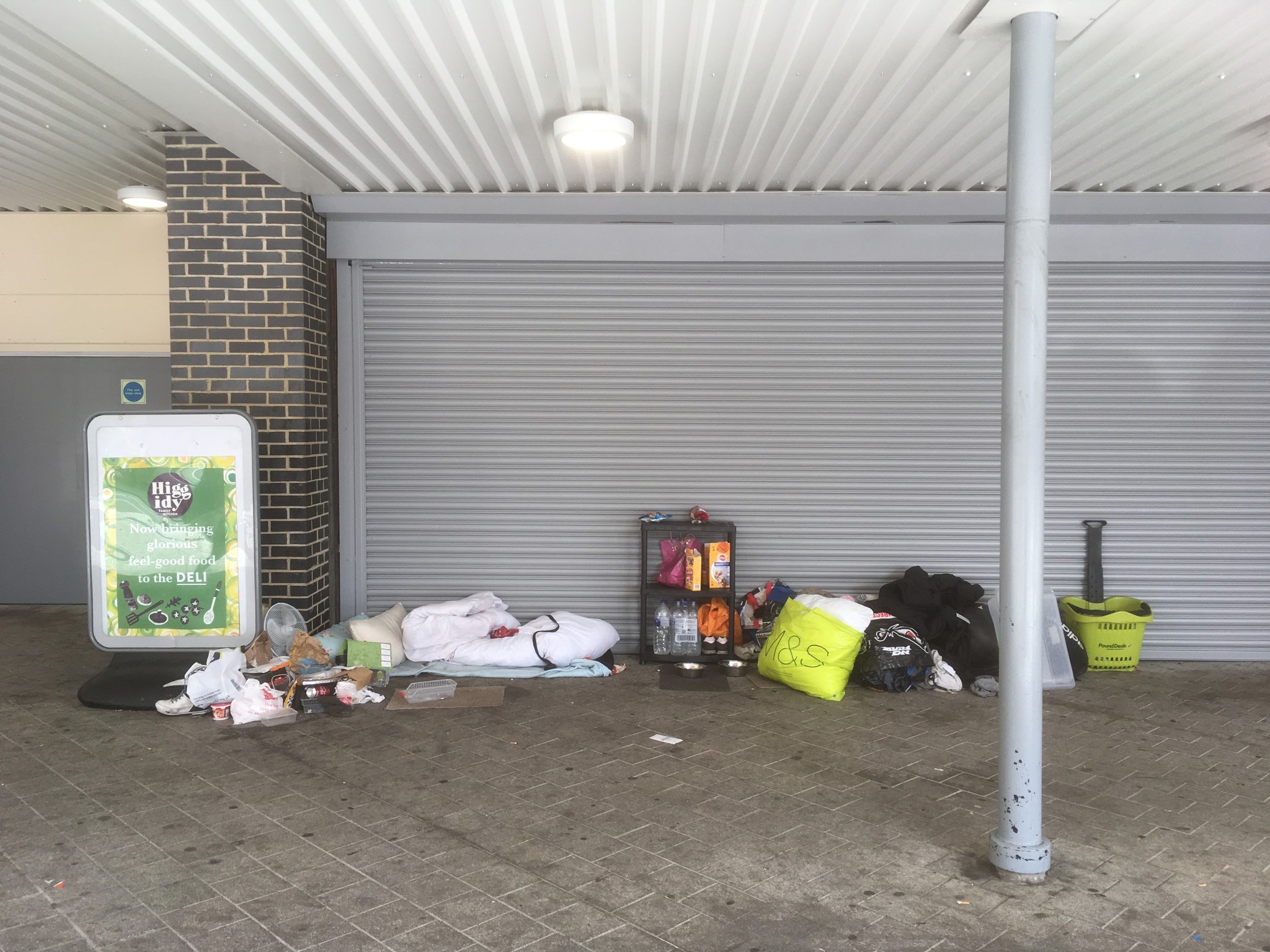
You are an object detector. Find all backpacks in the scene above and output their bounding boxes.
[1043,616,1089,681]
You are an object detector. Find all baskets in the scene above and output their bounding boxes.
[1058,596,1153,672]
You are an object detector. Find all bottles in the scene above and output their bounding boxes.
[670,597,702,656]
[653,600,672,655]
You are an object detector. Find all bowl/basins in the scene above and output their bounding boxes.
[717,659,749,677]
[674,662,707,678]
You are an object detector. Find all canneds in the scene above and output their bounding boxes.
[336,696,352,705]
[306,685,330,698]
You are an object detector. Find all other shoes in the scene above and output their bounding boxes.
[701,636,729,655]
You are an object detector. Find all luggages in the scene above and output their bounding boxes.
[955,602,999,677]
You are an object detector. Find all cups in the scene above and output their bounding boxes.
[211,703,231,721]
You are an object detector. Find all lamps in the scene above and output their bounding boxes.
[553,110,635,151]
[117,185,167,211]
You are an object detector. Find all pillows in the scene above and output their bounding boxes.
[349,602,408,667]
[312,613,370,667]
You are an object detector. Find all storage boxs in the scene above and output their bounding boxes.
[259,708,299,726]
[243,668,286,688]
[291,675,356,711]
[704,541,730,588]
[684,547,702,591]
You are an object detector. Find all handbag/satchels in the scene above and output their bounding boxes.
[850,611,935,694]
[654,534,703,588]
[758,597,865,701]
[697,597,742,645]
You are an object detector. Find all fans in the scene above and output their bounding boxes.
[263,603,308,657]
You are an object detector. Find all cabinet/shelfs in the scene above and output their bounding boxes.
[640,522,735,664]
